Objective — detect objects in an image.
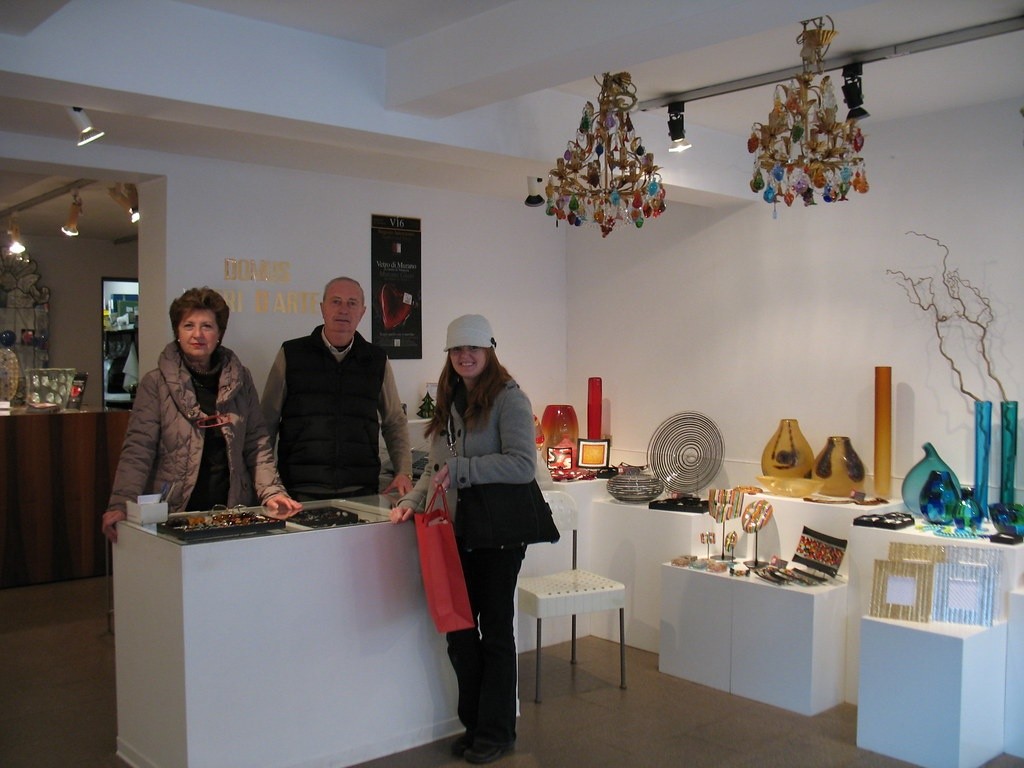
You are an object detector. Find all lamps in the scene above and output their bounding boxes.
[545,72,667,237]
[62,188,83,236]
[668,102,691,153]
[9,211,25,253]
[525,175,545,207]
[108,183,140,223]
[747,16,869,218]
[67,106,105,147]
[841,62,870,122]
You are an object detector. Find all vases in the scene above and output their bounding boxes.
[992,401,1024,524]
[969,400,996,521]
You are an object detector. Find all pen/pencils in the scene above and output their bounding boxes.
[159,482,175,503]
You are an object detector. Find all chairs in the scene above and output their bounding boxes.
[517,490,627,705]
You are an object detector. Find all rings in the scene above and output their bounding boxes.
[444,489,448,493]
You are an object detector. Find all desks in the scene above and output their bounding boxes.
[516,477,1023,768]
[107,495,467,768]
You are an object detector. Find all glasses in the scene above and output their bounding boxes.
[208,505,247,516]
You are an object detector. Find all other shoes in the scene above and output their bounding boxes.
[452,734,475,756]
[464,742,515,763]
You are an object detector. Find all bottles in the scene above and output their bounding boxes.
[607,467,662,504]
[542,405,579,470]
[953,486,980,532]
[919,471,958,525]
[762,419,814,478]
[901,443,962,516]
[812,437,865,497]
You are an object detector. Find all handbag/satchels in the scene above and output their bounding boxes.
[414,484,475,633]
[454,477,559,552]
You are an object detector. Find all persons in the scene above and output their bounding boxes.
[387,314,562,762]
[259,277,414,502]
[100,286,302,544]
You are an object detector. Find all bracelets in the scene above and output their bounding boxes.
[406,474,413,481]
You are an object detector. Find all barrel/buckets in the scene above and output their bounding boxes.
[67,368,89,409]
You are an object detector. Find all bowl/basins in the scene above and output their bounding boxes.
[989,504,1024,538]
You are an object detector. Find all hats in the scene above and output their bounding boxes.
[444,314,494,352]
